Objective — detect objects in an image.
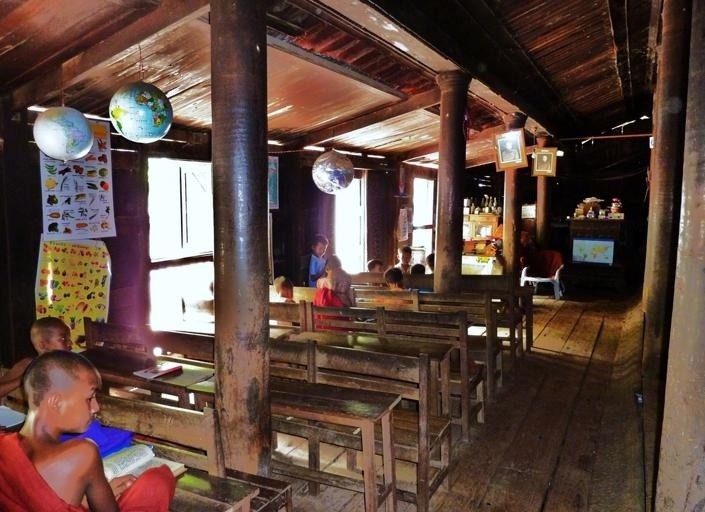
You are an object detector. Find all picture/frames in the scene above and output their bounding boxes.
[491,127,528,171]
[531,147,558,178]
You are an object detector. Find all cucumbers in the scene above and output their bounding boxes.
[86,182,98,190]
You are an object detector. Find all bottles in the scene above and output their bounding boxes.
[466,196,478,214]
[481,194,498,213]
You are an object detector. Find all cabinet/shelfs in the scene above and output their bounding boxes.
[459,212,504,240]
[561,209,628,304]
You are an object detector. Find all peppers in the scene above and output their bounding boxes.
[44,163,57,175]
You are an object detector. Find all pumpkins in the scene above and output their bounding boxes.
[45,178,59,189]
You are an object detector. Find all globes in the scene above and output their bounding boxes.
[311,148,356,196]
[108,80,174,144]
[32,106,94,160]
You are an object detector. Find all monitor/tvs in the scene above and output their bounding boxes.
[572,237,615,265]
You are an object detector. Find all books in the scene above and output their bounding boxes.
[97,438,189,484]
[134,359,182,383]
[0,404,27,429]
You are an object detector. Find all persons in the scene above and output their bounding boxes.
[502,140,520,163]
[0,316,102,406]
[270,276,297,326]
[427,253,435,274]
[408,263,433,292]
[316,256,345,289]
[0,350,176,512]
[374,267,413,312]
[366,258,385,274]
[312,269,353,335]
[539,155,552,171]
[308,234,330,285]
[394,247,412,275]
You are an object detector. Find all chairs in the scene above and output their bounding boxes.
[516,245,566,300]
